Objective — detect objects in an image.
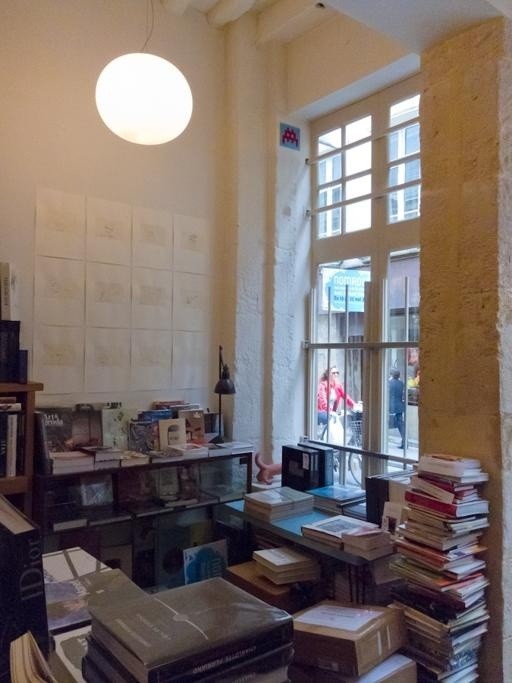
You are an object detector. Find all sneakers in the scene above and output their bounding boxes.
[397,444,408,448]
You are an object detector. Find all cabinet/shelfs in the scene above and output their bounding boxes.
[222,487,396,612]
[44,445,251,629]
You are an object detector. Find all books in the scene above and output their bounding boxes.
[0,396,489,683]
[1,260,21,320]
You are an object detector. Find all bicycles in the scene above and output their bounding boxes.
[318,398,363,487]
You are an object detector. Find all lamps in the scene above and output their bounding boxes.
[95,0,194,146]
[208,346,235,442]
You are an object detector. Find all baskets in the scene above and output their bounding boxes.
[348,420,361,436]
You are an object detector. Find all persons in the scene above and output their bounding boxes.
[316,364,355,424]
[407,367,420,387]
[390,367,406,448]
